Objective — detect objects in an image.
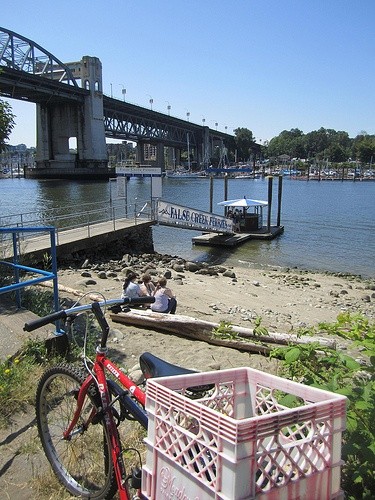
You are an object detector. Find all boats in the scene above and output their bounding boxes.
[165,170,206,180]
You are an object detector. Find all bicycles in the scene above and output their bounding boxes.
[21,295,346,500]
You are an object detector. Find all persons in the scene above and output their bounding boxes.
[120,272,150,308]
[151,277,177,314]
[227,209,242,233]
[139,273,156,306]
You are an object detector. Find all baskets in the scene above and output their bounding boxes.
[144,367,346,500]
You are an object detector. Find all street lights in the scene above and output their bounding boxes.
[183,108,190,121]
[109,80,113,98]
[122,86,126,102]
[213,120,218,131]
[200,114,205,126]
[145,94,154,110]
[163,100,171,115]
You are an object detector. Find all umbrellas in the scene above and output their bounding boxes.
[216,195,268,207]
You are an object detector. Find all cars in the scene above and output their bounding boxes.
[211,158,360,178]
